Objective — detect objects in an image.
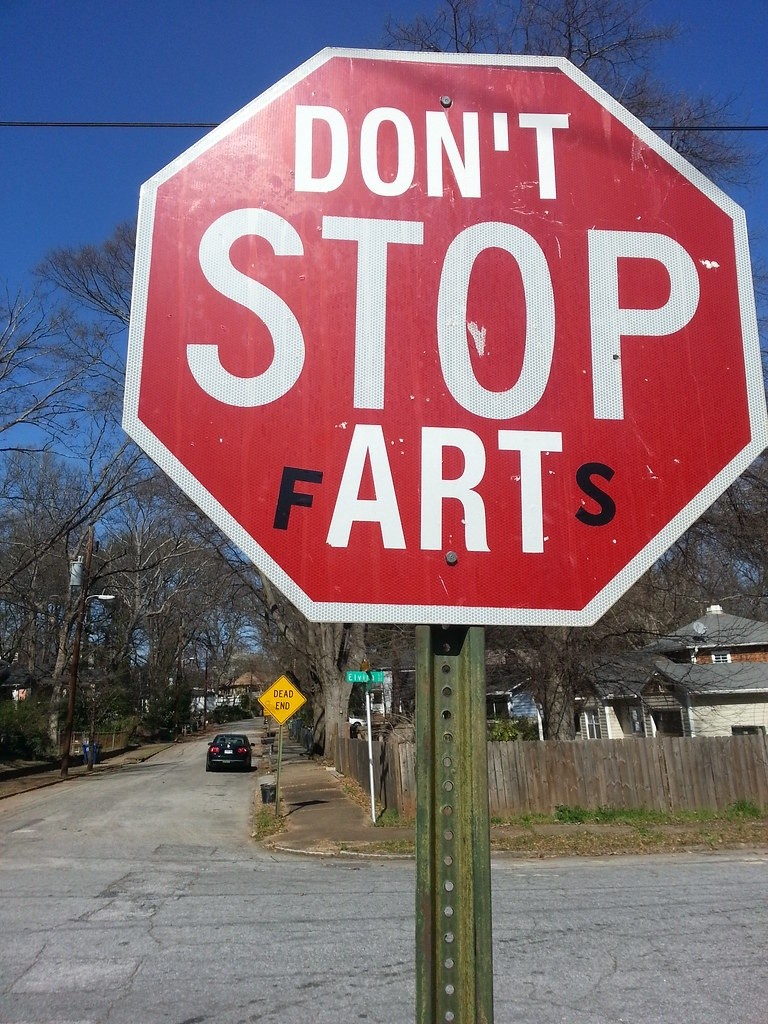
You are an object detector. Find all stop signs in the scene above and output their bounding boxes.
[118,48,768,626]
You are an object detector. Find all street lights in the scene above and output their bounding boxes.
[59,592,115,777]
[174,655,194,743]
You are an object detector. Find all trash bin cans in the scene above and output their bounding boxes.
[82,742,103,765]
[261,782,275,803]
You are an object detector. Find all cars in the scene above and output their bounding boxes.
[205,734,256,771]
[349,716,366,728]
